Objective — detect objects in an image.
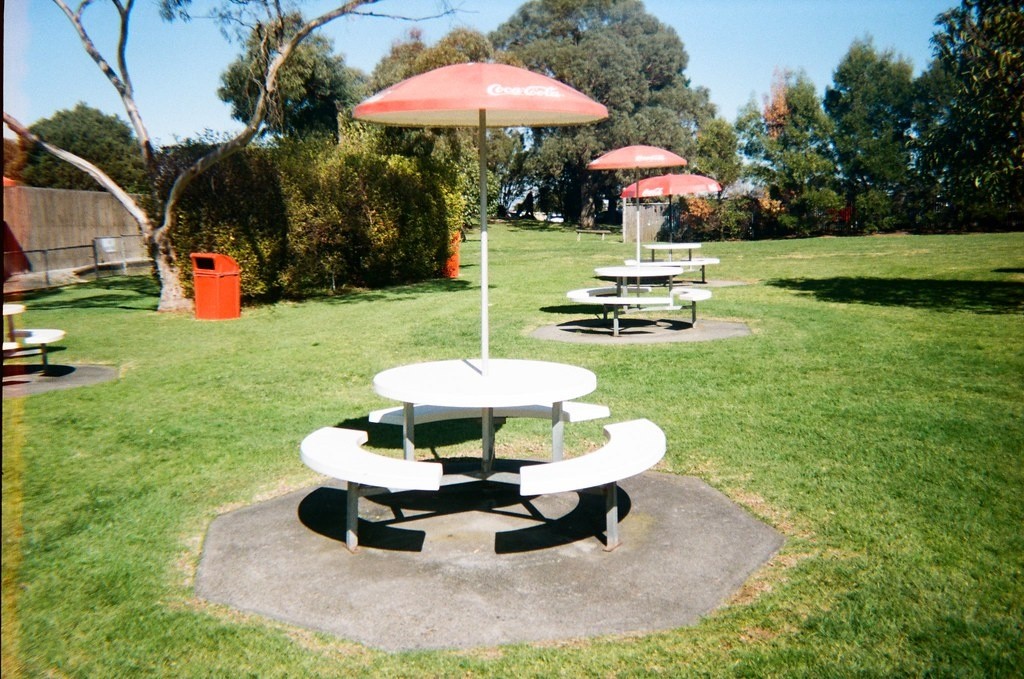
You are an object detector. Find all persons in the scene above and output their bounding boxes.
[524,191,533,216]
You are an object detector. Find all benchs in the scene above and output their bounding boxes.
[573,297,671,336]
[3,342,20,351]
[568,287,651,298]
[369,402,611,459]
[519,418,667,551]
[673,287,712,323]
[7,328,67,371]
[301,427,443,550]
[625,259,719,283]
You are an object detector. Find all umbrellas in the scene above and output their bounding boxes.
[587,145,722,309]
[354,61,610,473]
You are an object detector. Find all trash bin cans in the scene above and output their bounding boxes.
[190,252,240,320]
[442,231,460,279]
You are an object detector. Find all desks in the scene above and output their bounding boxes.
[373,358,598,462]
[595,267,683,297]
[3,305,25,342]
[641,243,702,269]
